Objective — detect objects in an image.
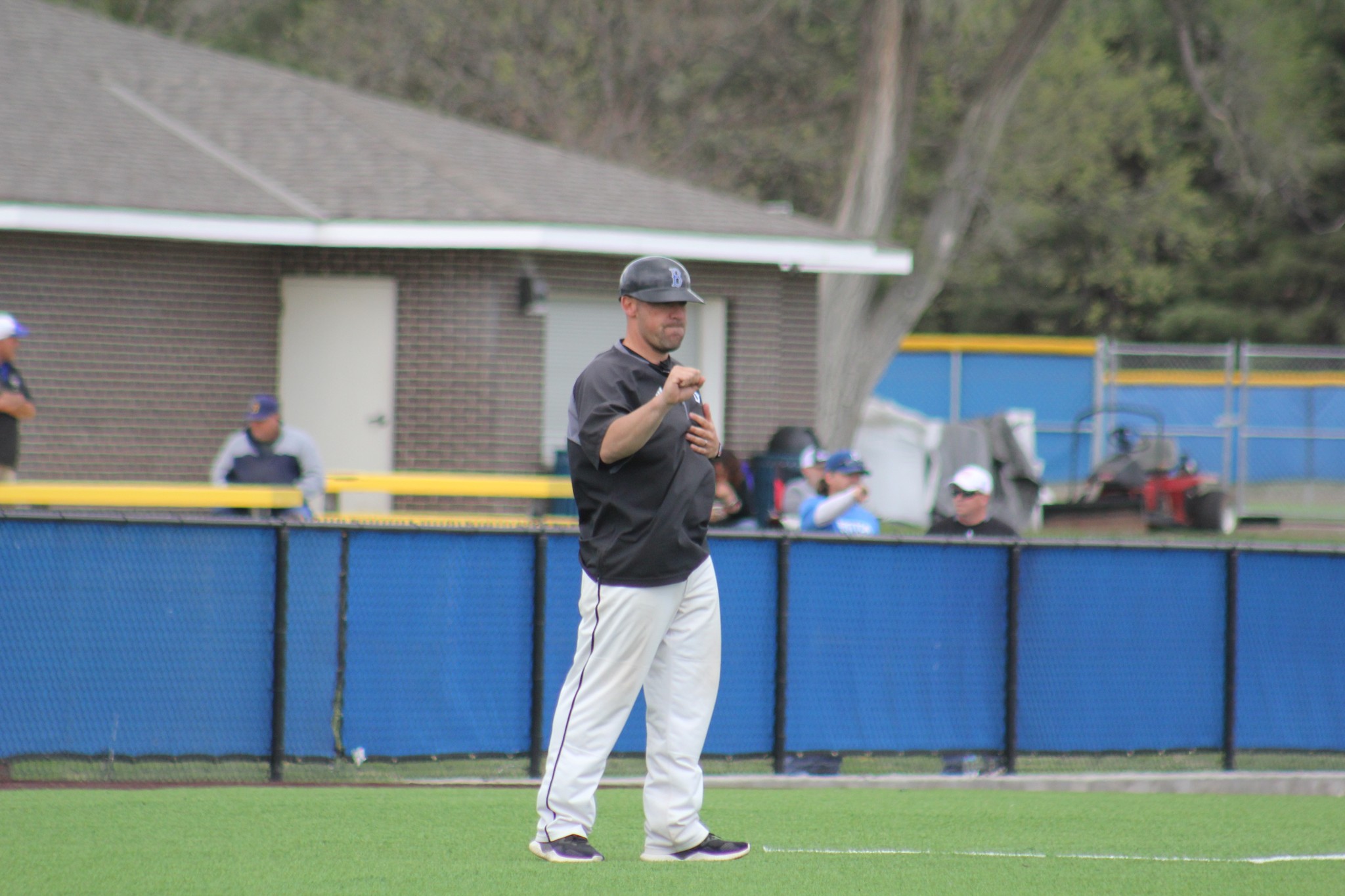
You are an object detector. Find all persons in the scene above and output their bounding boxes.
[210,397,325,520]
[710,450,746,525]
[0,309,37,482]
[529,257,747,867]
[925,465,1020,536]
[784,444,882,536]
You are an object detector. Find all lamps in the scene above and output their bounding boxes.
[519,276,553,320]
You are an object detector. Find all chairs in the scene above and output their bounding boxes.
[1129,437,1178,477]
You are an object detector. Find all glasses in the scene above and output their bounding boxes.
[951,490,976,497]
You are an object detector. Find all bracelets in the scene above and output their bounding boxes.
[717,443,722,461]
[712,507,723,511]
[726,499,740,507]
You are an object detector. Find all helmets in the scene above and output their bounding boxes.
[618,256,706,306]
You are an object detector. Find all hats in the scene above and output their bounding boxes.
[800,445,831,467]
[0,311,30,341]
[947,465,994,496]
[826,451,870,476]
[245,398,278,423]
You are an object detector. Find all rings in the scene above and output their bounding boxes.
[705,440,707,447]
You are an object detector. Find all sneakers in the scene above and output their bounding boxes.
[639,833,751,863]
[529,834,604,863]
